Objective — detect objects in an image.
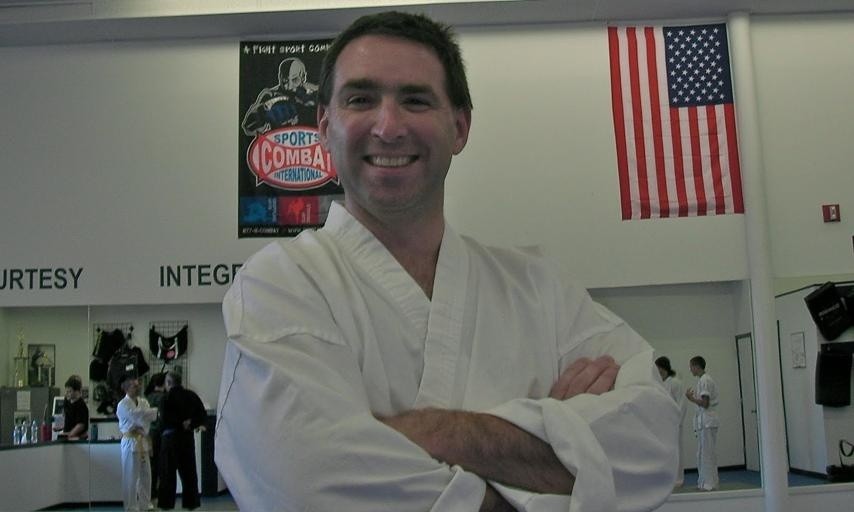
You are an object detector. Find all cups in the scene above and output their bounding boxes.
[91,423,98,443]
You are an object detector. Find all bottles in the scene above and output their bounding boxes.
[14,420,52,446]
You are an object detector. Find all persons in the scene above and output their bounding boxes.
[216,13,685,512]
[241,54,321,136]
[114,369,209,512]
[654,354,722,495]
[60,375,89,440]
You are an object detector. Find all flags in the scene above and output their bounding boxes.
[606,19,747,220]
